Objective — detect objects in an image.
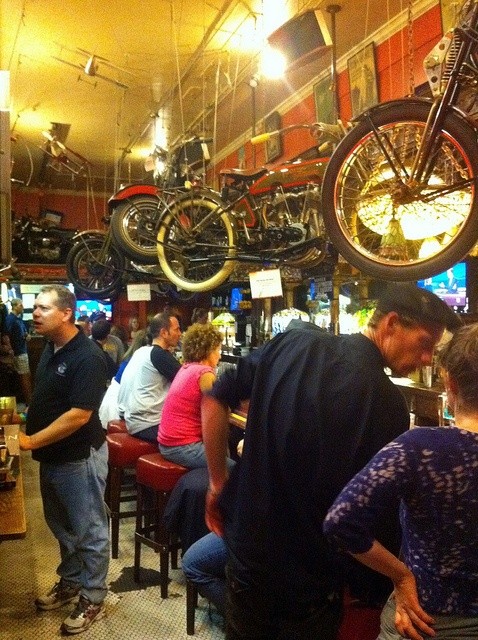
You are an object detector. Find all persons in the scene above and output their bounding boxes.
[5,296,36,408]
[322,320,478,640]
[117,330,153,420]
[126,313,141,348]
[123,306,182,444]
[181,507,241,620]
[157,324,239,470]
[201,285,462,640]
[89,320,125,368]
[97,329,149,430]
[18,283,115,634]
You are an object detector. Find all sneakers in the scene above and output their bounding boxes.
[35,581,74,610]
[61,601,107,634]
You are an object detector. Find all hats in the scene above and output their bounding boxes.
[376,281,465,331]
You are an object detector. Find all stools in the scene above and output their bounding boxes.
[134,454,194,597]
[105,432,159,560]
[107,419,125,432]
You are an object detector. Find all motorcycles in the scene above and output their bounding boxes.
[155,119,399,293]
[320,0,477,282]
[107,135,244,265]
[11,215,77,263]
[66,216,210,305]
